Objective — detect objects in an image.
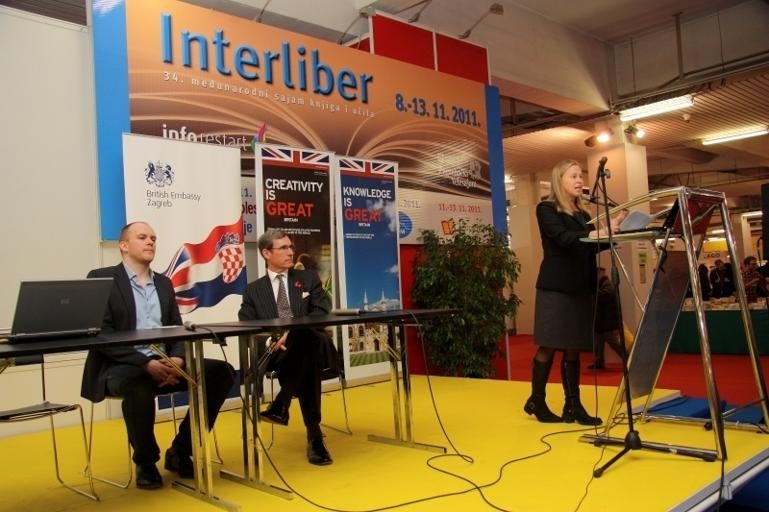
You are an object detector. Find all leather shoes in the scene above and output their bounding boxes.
[165,449,194,478]
[307,438,332,465]
[135,464,163,490]
[260,404,289,426]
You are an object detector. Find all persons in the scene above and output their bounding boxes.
[583,262,636,370]
[681,253,768,305]
[520,159,629,427]
[236,228,345,465]
[80,222,234,491]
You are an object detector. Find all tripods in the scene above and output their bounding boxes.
[591,157,717,478]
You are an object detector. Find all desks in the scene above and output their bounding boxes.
[669,308,769,356]
[0,325,263,512]
[203,306,470,500]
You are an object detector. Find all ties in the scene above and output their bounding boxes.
[277,274,294,319]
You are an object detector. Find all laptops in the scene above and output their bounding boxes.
[0,277,114,344]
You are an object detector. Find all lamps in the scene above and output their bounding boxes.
[619,94,695,121]
[458,1,505,39]
[584,120,643,151]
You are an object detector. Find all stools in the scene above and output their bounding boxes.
[253,334,352,452]
[84,381,224,490]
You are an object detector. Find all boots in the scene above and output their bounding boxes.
[524,357,562,422]
[561,358,603,425]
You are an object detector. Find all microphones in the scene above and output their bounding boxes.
[184,321,196,330]
[581,193,610,205]
[331,309,360,315]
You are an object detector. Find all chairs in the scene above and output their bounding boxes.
[0,330,103,502]
